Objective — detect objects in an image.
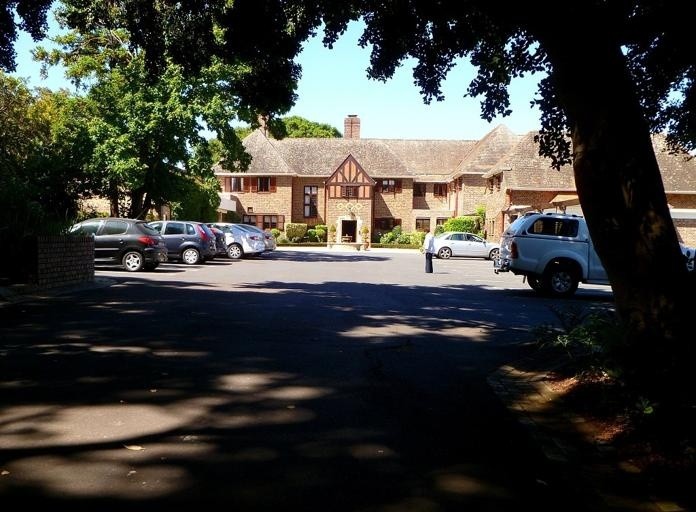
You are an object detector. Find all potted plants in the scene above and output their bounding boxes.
[361,226,369,249]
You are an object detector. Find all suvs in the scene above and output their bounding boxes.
[493,212,696,297]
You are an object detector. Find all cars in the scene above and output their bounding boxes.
[208,225,225,259]
[235,224,276,256]
[147,220,211,265]
[67,217,169,273]
[426,231,500,261]
[204,222,266,259]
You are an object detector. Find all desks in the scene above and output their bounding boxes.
[342,236,352,243]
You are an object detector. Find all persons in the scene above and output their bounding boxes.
[423,227,434,273]
